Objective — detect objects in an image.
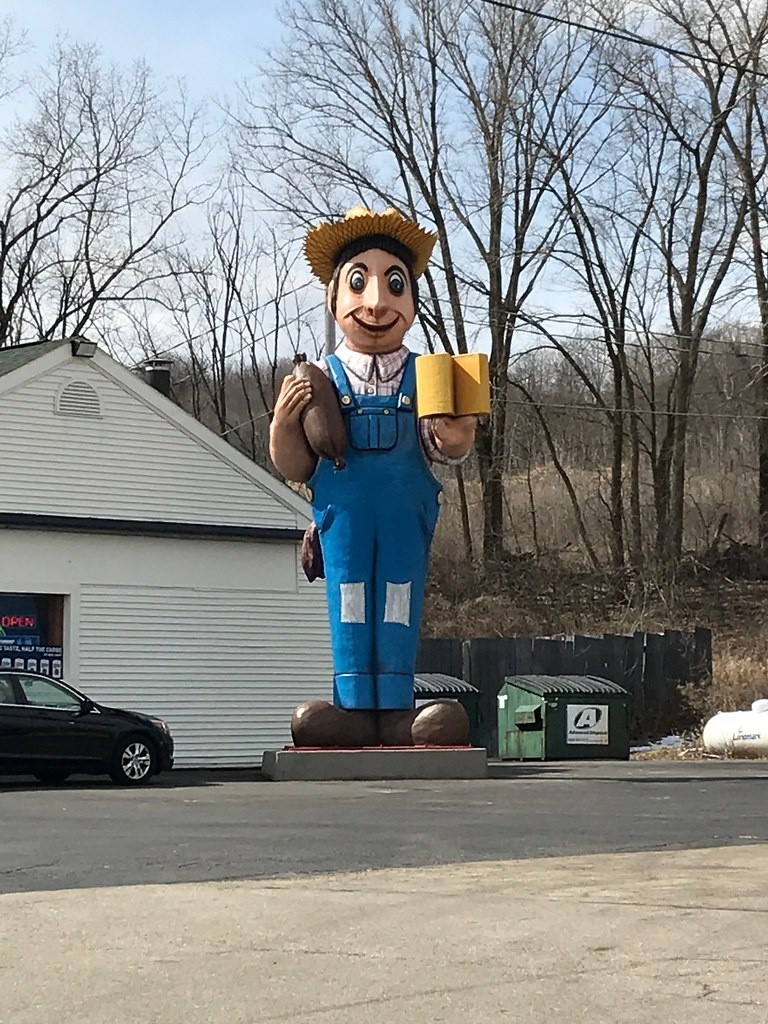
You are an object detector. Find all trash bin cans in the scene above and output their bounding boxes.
[496,674,632,761]
[414,672,482,749]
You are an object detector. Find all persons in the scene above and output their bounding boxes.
[269,206,477,749]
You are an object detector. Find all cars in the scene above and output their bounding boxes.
[0,666,174,788]
